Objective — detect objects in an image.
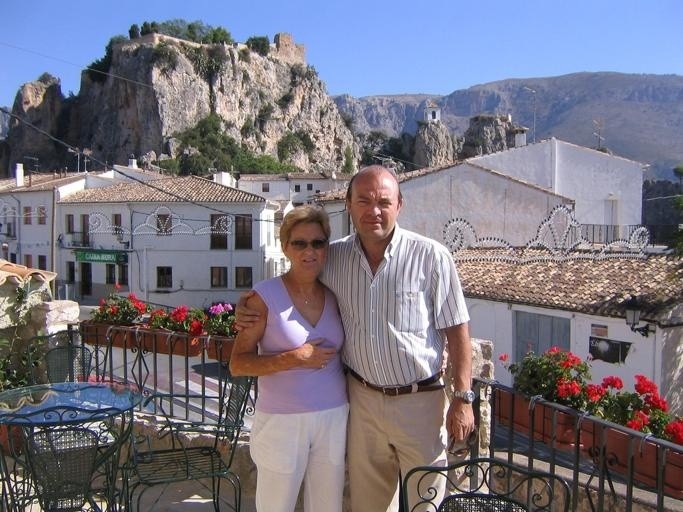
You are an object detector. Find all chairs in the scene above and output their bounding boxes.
[393,454,575,512]
[1,404,140,512]
[26,327,120,512]
[121,345,259,511]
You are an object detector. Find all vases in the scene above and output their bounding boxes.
[124,324,208,358]
[79,318,139,350]
[206,335,238,364]
[577,414,683,503]
[493,385,584,452]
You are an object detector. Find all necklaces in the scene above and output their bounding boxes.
[283,274,320,309]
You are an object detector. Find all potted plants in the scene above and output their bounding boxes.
[1,279,45,457]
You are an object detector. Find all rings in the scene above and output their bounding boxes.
[321,364,325,369]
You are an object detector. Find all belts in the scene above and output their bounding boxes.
[348,368,445,396]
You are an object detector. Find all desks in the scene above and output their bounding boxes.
[1,379,143,512]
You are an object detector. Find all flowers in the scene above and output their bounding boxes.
[90,280,147,326]
[141,304,208,338]
[498,345,586,406]
[577,374,683,447]
[203,299,241,337]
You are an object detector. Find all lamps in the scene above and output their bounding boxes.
[623,294,654,340]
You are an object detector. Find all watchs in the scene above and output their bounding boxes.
[453,389,475,404]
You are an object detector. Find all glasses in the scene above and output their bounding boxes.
[290,239,326,249]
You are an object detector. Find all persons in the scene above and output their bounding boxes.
[226,205,347,511]
[233,163,477,511]
